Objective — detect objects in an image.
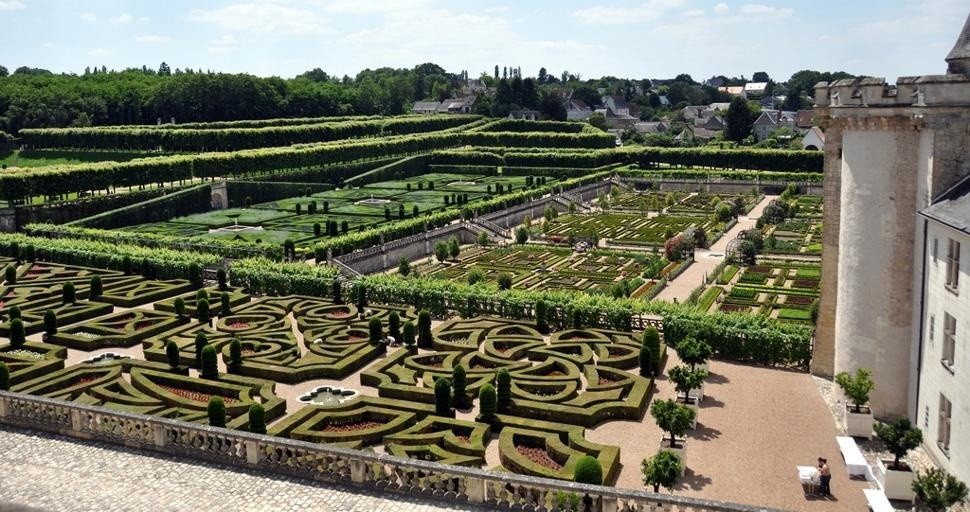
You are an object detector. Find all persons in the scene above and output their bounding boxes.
[818,457,825,495]
[818,458,831,495]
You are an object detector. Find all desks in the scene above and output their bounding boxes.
[796,466,821,501]
[837,436,868,478]
[863,489,894,512]
